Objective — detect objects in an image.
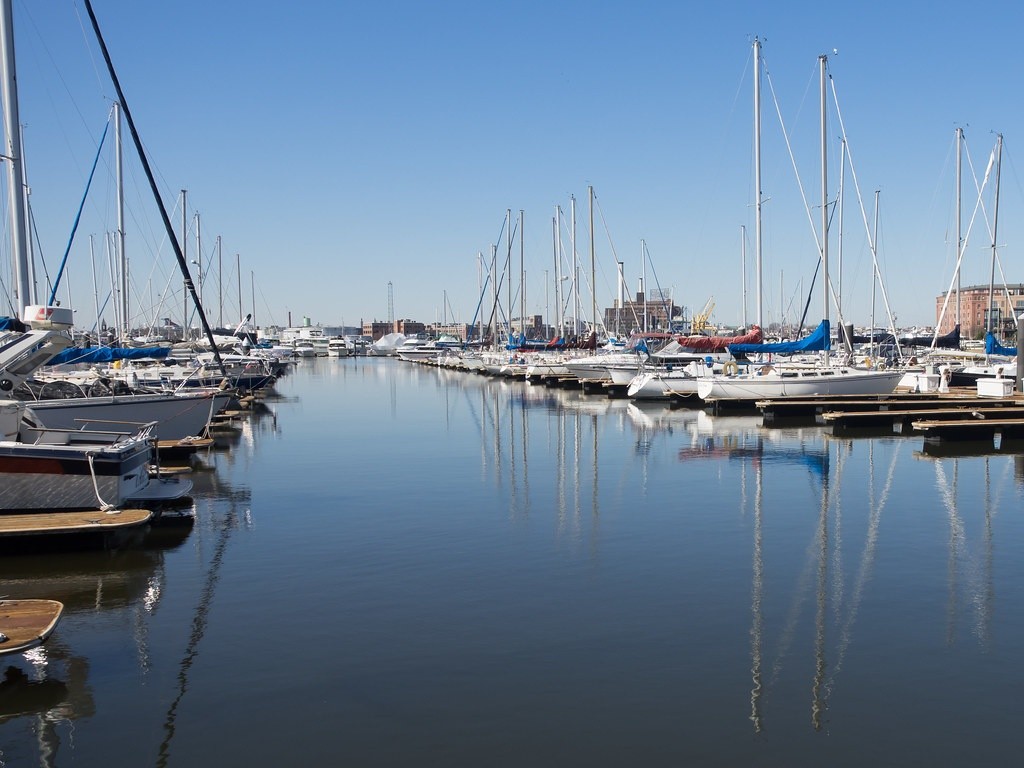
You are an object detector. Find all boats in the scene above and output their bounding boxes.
[0,596,64,656]
[0,508,155,536]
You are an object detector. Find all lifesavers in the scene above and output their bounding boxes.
[723,361,738,375]
[260,343,273,348]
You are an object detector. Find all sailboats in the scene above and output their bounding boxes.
[0,0,1024,511]
